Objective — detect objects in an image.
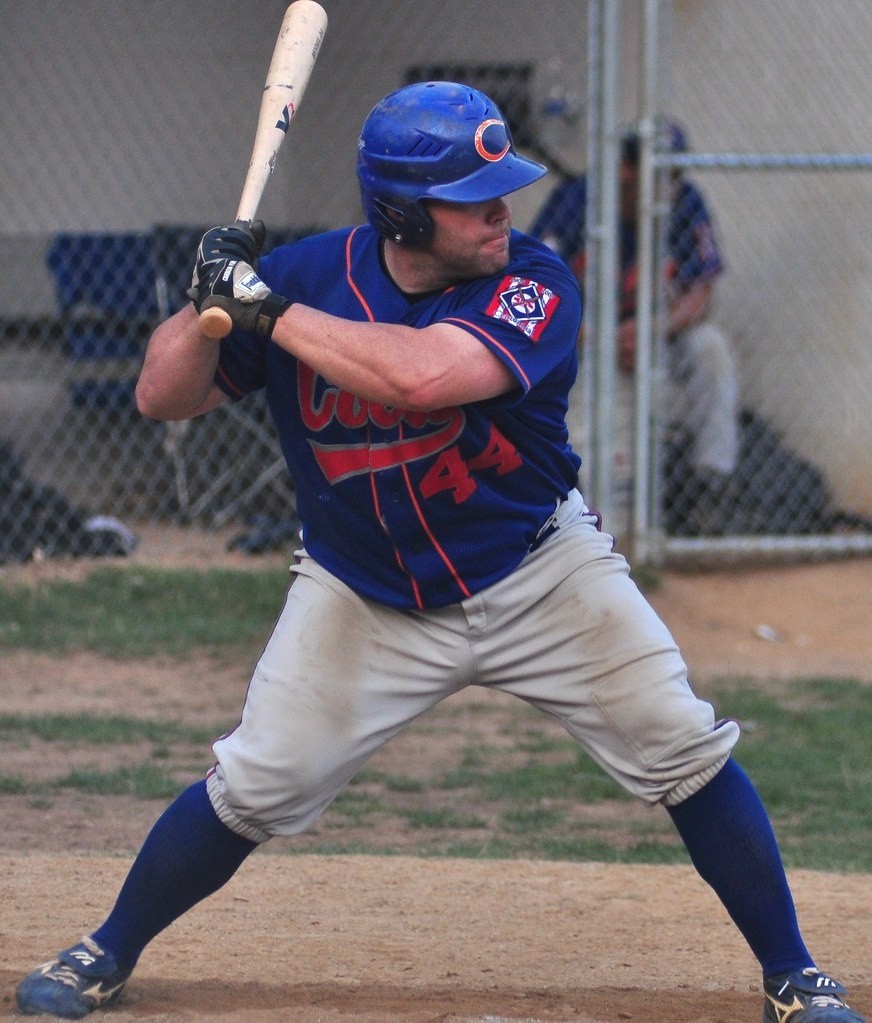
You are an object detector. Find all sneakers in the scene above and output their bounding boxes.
[764,964,867,1022]
[15,934,126,1018]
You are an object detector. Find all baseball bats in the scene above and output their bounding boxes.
[195,1,331,343]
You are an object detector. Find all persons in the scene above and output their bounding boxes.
[529,115,734,530]
[14,81,859,1022]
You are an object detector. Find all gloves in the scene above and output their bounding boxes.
[192,220,265,287]
[183,261,293,353]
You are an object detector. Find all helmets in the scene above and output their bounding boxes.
[624,113,686,178]
[355,79,547,246]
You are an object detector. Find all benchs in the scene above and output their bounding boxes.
[46,232,330,521]
